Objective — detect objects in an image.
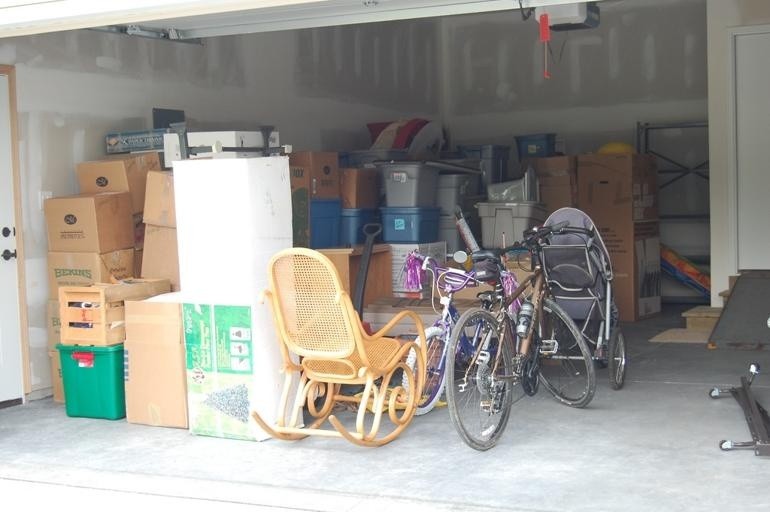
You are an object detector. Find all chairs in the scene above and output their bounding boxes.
[248,245,429,451]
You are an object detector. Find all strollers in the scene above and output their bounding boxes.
[522,205,628,389]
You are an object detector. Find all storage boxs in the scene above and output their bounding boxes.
[38,111,308,446]
[289,109,663,345]
